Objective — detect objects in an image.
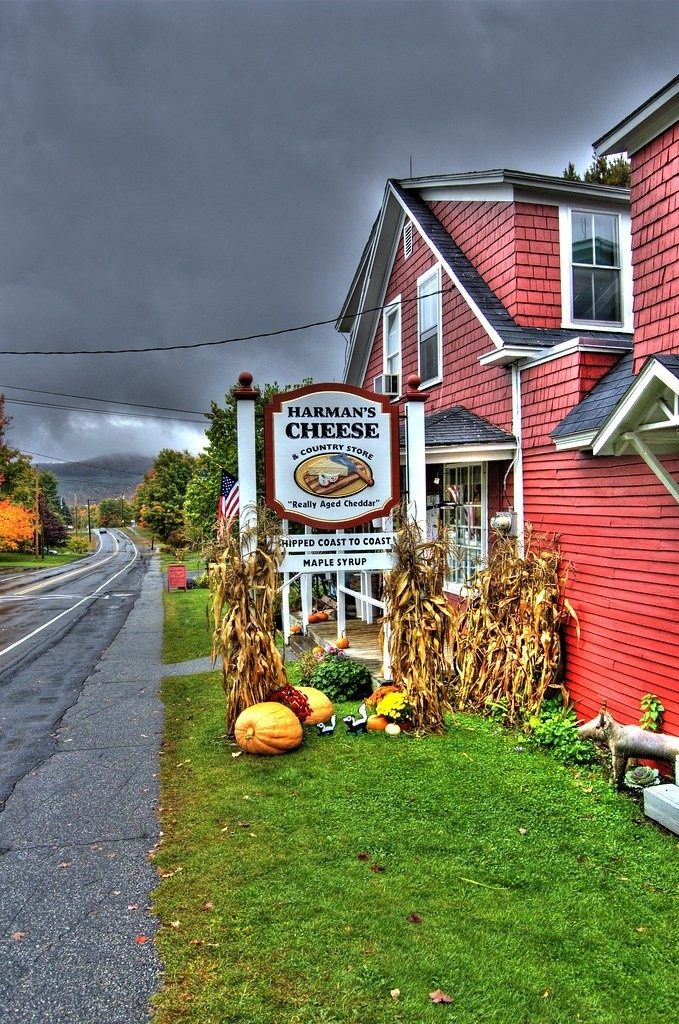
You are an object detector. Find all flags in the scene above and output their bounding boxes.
[217,469,239,542]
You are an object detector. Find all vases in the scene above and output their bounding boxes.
[399,723,411,730]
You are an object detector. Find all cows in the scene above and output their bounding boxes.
[577,698,679,791]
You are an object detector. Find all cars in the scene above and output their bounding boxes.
[64,525,74,531]
[40,547,57,556]
[99,527,107,533]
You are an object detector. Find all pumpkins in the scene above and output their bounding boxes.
[288,611,349,655]
[234,686,411,755]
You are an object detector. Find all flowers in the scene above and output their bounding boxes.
[368,686,413,723]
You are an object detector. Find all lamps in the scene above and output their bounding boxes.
[434,472,451,485]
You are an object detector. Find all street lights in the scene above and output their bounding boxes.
[87,497,98,542]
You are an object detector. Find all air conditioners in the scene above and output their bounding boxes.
[374,374,399,397]
